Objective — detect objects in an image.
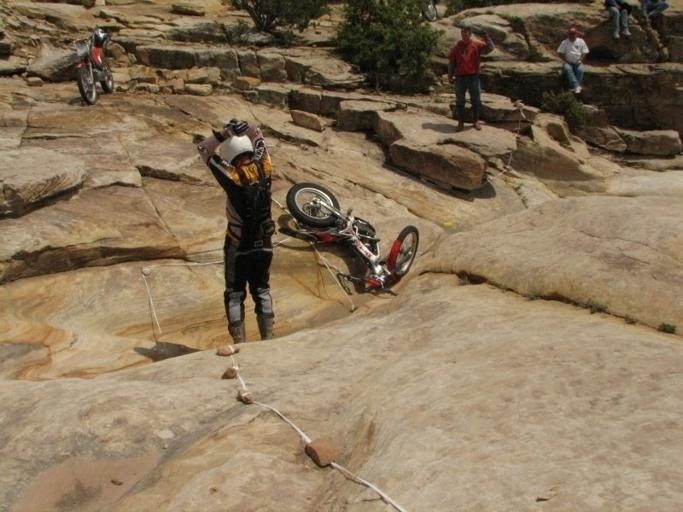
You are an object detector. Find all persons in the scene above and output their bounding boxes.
[446,25,495,132]
[557,27,589,94]
[604,0,669,39]
[195,116,280,344]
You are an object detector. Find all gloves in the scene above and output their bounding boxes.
[227,122,249,134]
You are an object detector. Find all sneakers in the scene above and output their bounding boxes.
[576,87,582,95]
[614,31,621,38]
[622,29,631,36]
[456,123,464,132]
[473,121,482,131]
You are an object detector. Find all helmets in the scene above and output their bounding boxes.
[220,133,253,163]
[93,29,108,47]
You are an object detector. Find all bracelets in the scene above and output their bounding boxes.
[211,127,232,143]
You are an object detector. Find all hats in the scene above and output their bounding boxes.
[568,28,577,33]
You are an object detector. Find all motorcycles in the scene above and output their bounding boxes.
[278,182,419,296]
[72,29,114,105]
